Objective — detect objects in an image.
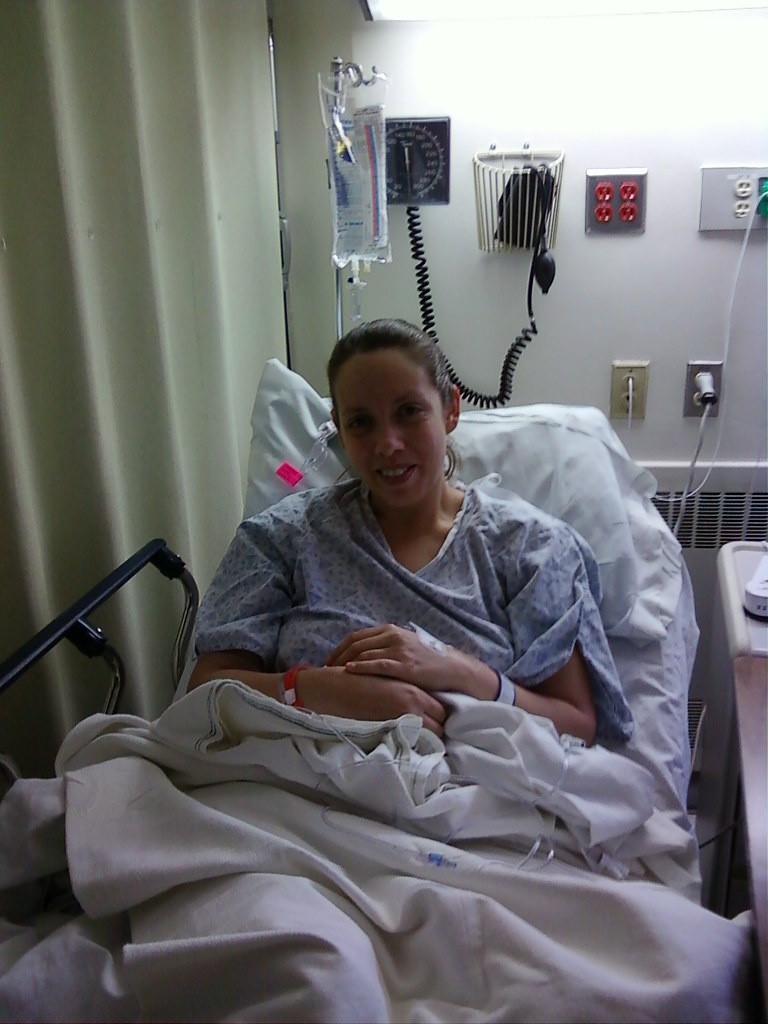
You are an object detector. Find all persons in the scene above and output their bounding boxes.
[0,316,746,1024]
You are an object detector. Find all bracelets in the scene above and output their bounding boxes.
[494,668,516,706]
[279,663,310,708]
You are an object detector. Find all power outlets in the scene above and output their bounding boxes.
[585,168,648,234]
[610,360,649,419]
[683,361,723,417]
[698,167,768,232]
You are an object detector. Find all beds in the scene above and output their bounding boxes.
[1,357,768,1024]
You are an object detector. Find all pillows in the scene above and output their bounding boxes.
[242,357,683,644]
